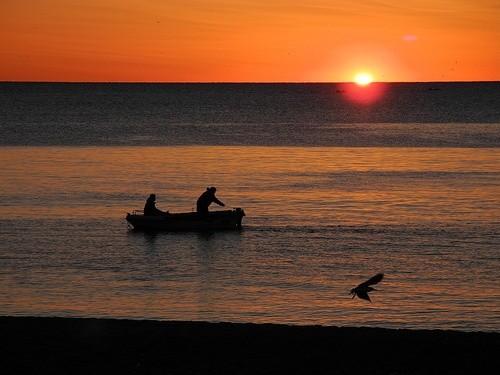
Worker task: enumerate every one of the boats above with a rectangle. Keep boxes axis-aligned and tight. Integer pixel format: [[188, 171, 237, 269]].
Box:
[[126, 204, 245, 235]]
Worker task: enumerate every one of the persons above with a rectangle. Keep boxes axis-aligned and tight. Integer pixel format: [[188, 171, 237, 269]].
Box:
[[196, 186, 225, 213], [144, 194, 161, 216]]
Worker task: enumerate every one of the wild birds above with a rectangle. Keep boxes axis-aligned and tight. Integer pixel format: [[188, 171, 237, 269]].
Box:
[[348, 272, 385, 304]]
